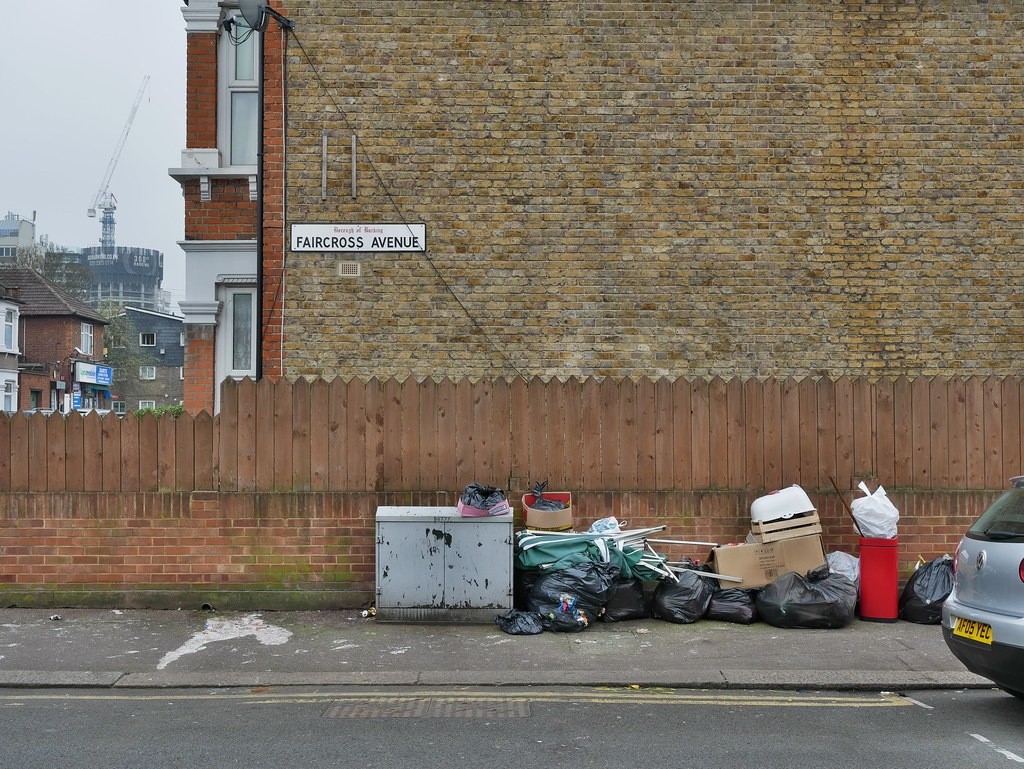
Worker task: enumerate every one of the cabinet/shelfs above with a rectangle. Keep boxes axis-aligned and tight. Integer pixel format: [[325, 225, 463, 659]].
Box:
[[374, 506, 514, 625]]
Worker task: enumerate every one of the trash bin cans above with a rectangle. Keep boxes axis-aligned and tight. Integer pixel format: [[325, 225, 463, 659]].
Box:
[[859, 535, 899, 623]]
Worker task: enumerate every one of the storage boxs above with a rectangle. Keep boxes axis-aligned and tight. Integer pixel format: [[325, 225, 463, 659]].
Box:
[[710, 534, 828, 590], [521, 492, 573, 533]]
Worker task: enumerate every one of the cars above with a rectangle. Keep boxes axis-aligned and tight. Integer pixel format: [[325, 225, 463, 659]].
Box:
[[940, 473, 1024, 701]]
